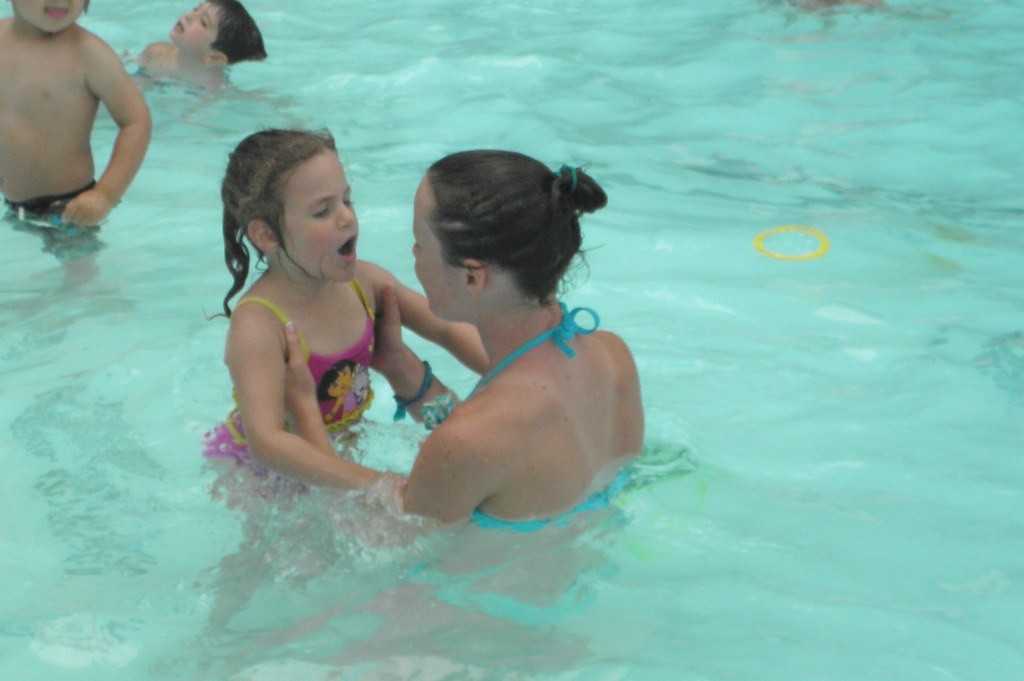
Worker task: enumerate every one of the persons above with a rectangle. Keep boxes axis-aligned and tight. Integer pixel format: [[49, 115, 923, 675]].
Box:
[[283, 149, 647, 521], [140, 0, 268, 88], [0, 0, 152, 228], [219, 128, 489, 494]]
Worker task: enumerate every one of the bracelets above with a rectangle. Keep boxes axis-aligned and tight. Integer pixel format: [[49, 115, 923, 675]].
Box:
[[394, 361, 433, 420]]
